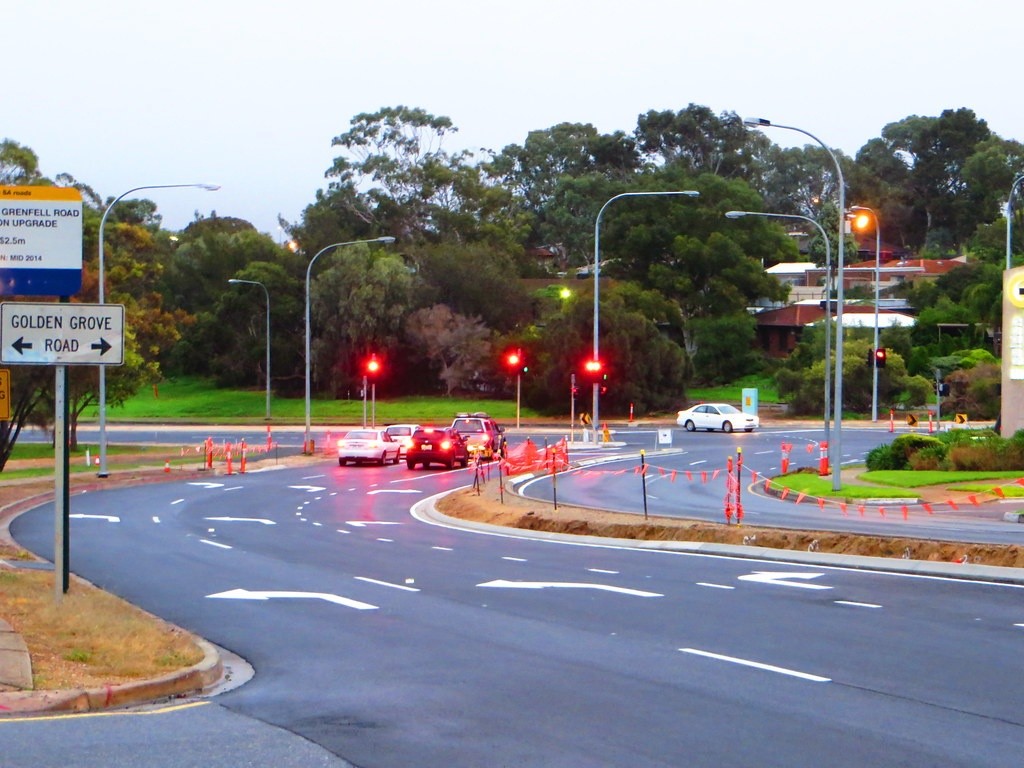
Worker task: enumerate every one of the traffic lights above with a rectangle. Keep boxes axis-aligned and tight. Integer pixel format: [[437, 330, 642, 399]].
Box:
[[602, 373, 607, 381], [598, 386, 608, 396], [876, 349, 887, 369], [574, 388, 579, 398], [865, 348, 873, 367], [522, 366, 529, 373], [508, 354, 521, 374], [586, 358, 602, 376], [366, 359, 382, 381]]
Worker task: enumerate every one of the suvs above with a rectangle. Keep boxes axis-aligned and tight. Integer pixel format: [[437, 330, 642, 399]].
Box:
[[405, 426, 471, 471], [450, 412, 507, 463]]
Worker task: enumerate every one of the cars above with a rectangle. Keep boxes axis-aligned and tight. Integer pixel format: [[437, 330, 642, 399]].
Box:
[[385, 424, 424, 456], [337, 429, 402, 466], [675, 402, 761, 433]]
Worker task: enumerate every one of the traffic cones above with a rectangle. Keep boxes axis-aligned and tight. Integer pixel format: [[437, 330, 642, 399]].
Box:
[[163, 458, 172, 473], [94, 453, 99, 466]]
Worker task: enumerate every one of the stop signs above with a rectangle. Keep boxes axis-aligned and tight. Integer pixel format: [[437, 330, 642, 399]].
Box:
[[1, 302, 126, 368]]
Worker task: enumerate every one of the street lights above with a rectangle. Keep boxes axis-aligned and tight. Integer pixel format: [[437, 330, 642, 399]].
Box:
[[591, 190, 700, 447], [851, 205, 879, 423], [745, 115, 844, 493], [304, 236, 397, 443], [99, 181, 222, 485], [228, 277, 275, 421], [725, 209, 829, 462]]
